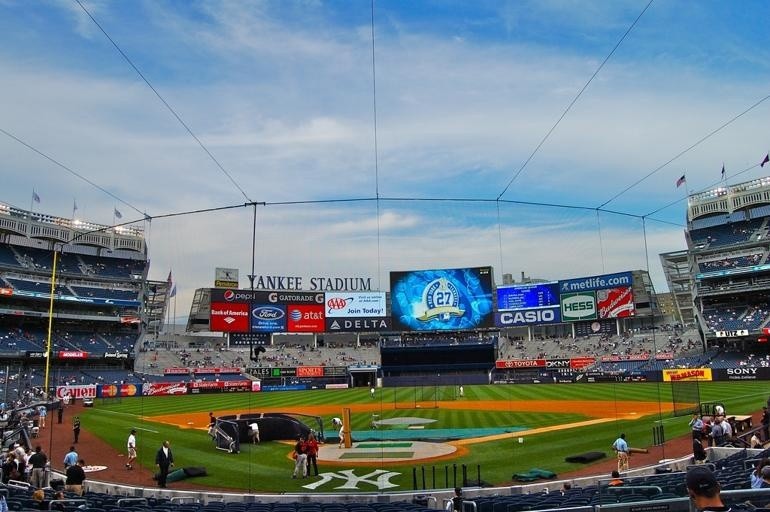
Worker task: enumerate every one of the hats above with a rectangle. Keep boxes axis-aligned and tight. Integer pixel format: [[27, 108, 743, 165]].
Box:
[[688, 467, 716, 489], [455, 488, 462, 496], [763, 466, 770, 474]]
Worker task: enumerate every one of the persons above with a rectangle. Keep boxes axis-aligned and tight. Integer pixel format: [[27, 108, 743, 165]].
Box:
[[0, 225, 770, 512]]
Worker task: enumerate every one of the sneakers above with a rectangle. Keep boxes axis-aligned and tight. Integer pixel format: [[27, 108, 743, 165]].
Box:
[[126, 463, 133, 470]]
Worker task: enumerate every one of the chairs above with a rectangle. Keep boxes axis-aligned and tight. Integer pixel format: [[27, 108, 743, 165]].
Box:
[[687, 214, 769, 371], [0, 241, 253, 387], [590, 359, 766, 372], [380, 335, 495, 349]]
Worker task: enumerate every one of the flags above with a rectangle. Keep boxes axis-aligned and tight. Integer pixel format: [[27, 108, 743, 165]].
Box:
[[74, 199, 78, 212], [677, 176, 685, 188], [33, 193, 40, 203], [761, 155, 769, 168], [721, 166, 724, 180], [115, 210, 122, 219], [145, 215, 150, 222]]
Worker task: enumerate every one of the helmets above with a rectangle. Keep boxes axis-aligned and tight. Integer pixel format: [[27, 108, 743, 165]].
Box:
[[131, 430, 136, 433]]
